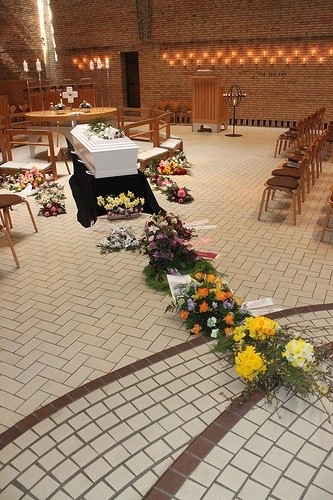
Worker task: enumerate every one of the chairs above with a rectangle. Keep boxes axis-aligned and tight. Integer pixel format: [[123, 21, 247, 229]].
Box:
[[0, 194, 38, 233], [258, 105, 333, 244]]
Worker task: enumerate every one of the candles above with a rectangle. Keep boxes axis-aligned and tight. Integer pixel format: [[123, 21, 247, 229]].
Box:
[[162, 49, 333, 66], [23, 59, 29, 71], [36, 59, 42, 70], [90, 57, 110, 70]]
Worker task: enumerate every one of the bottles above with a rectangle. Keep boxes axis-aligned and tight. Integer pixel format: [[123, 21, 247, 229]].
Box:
[[49, 102, 59, 115]]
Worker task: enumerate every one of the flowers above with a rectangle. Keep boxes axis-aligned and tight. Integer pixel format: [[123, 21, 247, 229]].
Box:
[[142, 151, 195, 204], [141, 214, 224, 292], [216, 316, 333, 424], [166, 267, 255, 337], [96, 226, 144, 254], [97, 191, 145, 220], [1, 167, 67, 218], [87, 121, 125, 139]]
